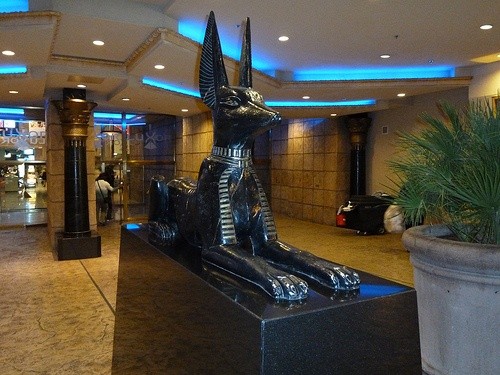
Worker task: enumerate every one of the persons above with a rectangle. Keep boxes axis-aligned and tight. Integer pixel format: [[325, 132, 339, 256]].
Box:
[[399, 183, 426, 252], [93, 170, 123, 229]]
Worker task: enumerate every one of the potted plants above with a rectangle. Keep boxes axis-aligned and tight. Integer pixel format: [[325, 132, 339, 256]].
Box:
[[378, 88, 500, 375]]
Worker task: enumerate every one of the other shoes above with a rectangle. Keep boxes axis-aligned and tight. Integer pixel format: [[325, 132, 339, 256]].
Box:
[[100, 222, 109, 225]]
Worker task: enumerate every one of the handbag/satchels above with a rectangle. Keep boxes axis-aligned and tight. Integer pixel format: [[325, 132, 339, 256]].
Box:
[[103, 197, 111, 204]]
